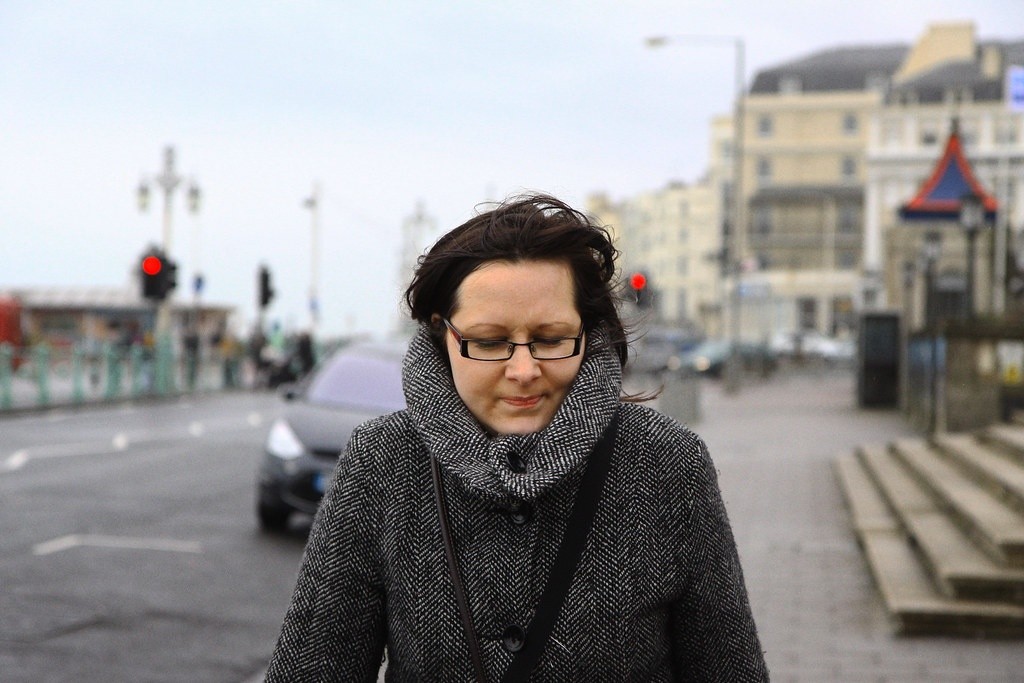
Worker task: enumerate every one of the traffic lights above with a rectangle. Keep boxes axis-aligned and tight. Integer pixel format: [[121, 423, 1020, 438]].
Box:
[[627, 269, 652, 308], [140, 247, 170, 303]]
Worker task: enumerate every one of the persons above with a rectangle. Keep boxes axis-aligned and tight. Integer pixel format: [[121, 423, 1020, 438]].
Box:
[[264, 191, 769, 683]]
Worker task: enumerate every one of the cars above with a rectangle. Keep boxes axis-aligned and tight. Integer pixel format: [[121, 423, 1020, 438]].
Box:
[[255, 336, 409, 531]]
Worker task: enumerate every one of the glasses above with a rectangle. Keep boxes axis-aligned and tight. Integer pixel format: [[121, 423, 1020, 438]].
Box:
[[442, 318, 587, 362]]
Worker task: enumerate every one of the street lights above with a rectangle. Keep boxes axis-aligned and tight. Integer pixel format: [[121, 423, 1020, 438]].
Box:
[[960, 191, 984, 333], [644, 34, 749, 396], [135, 148, 201, 402]]
